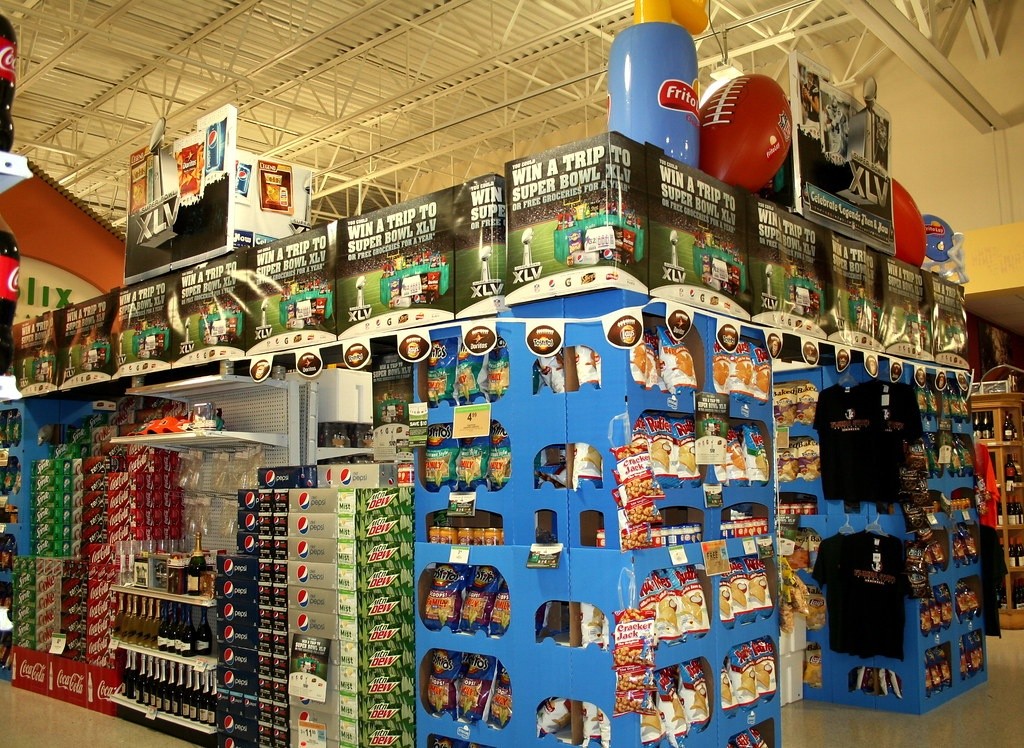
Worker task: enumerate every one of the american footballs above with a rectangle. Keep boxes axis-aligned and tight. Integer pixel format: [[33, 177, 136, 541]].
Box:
[[699, 72, 793, 189], [891, 176, 928, 269]]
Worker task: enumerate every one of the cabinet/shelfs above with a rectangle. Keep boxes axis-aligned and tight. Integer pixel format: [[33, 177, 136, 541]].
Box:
[[972, 392, 1024, 630], [108, 378, 301, 748]]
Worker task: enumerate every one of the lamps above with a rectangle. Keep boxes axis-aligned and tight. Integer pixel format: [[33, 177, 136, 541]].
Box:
[[699, 30, 745, 109]]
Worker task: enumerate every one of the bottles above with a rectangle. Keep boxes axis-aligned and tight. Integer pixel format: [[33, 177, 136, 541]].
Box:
[[995, 578, 1024, 609], [973, 412, 981, 440], [1000, 537, 1024, 567], [127, 652, 137, 698], [113, 594, 212, 657], [188, 531, 206, 594], [151, 658, 217, 725], [144, 656, 153, 705], [122, 650, 131, 695], [1003, 415, 1012, 440], [997, 495, 1024, 524], [1013, 454, 1022, 481], [983, 412, 991, 438], [135, 654, 146, 704], [1006, 453, 1015, 481]]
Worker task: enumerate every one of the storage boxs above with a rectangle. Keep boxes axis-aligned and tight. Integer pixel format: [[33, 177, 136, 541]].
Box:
[[11, 395, 194, 672], [286, 367, 373, 425], [779, 612, 807, 655], [779, 651, 805, 707], [11, 130, 970, 392], [214, 462, 414, 748]]
